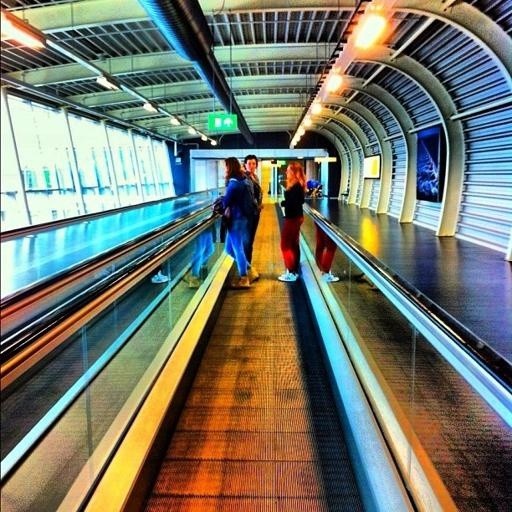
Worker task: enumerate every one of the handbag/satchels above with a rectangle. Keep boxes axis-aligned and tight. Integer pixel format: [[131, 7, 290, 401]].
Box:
[[212, 197, 231, 218]]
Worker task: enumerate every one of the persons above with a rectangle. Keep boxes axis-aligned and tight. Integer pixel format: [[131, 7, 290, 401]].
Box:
[[306, 179, 322, 199], [188, 221, 216, 290], [313, 221, 340, 283], [151, 265, 169, 284], [244, 154, 264, 265], [277, 161, 308, 283], [240, 165, 246, 171], [219, 157, 261, 290]]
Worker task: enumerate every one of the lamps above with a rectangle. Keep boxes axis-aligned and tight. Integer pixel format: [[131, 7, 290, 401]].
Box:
[[97, 76, 124, 93], [1, 8, 46, 52], [289, 0, 386, 149], [143, 101, 218, 146]]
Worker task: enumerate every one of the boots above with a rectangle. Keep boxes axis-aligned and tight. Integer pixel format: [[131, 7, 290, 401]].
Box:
[[185, 265, 208, 287], [229, 266, 260, 288]]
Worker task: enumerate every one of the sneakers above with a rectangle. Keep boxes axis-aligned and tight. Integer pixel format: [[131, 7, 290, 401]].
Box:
[[151, 270, 169, 284], [277, 270, 299, 282], [323, 272, 339, 282]]
